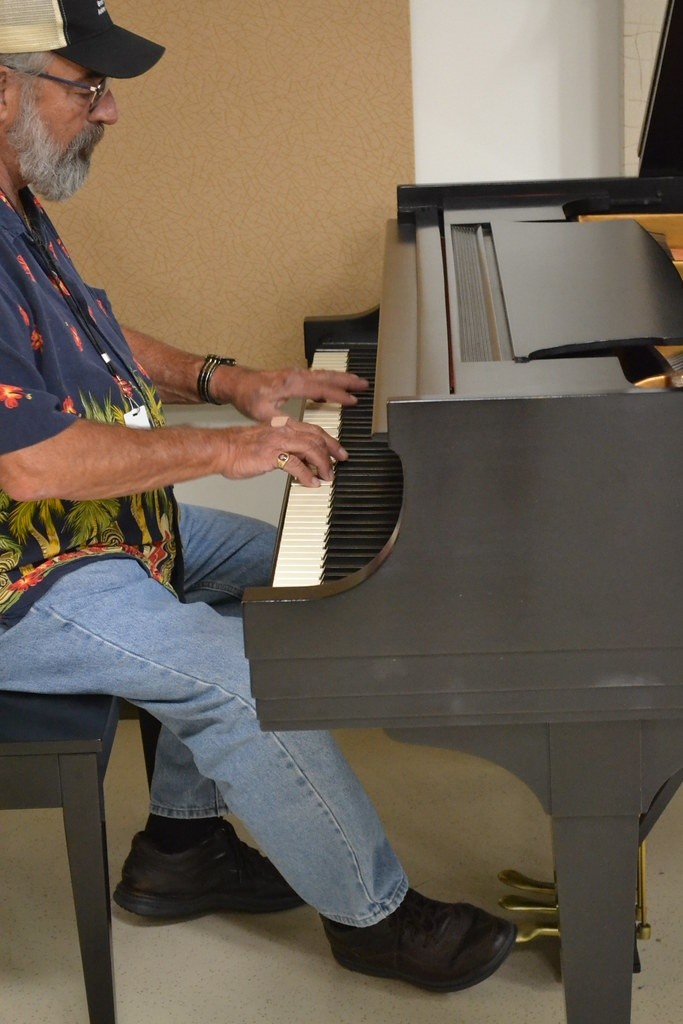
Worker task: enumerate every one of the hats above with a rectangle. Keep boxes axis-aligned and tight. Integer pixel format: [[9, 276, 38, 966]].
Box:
[[0, 0, 166, 78]]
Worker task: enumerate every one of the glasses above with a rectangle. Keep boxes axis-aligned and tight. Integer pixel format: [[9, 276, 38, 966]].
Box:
[[3, 64, 111, 114]]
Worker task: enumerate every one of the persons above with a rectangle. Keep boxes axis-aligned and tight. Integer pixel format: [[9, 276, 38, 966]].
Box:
[[0, 0, 518, 993]]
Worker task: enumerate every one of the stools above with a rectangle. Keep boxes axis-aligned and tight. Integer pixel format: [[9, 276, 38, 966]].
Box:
[[0, 690, 165, 1024]]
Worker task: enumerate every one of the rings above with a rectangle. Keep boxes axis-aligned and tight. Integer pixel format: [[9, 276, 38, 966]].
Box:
[[271, 416, 290, 427], [277, 452, 289, 469]]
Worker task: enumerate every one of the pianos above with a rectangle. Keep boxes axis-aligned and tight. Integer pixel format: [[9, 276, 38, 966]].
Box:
[[240, 0, 683, 1024]]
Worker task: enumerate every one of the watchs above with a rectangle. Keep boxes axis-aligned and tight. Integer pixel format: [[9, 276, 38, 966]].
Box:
[[197, 355, 236, 406]]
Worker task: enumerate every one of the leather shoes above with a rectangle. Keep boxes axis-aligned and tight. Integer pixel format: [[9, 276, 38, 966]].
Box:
[[319, 888, 517, 993], [112, 813, 307, 917]]
